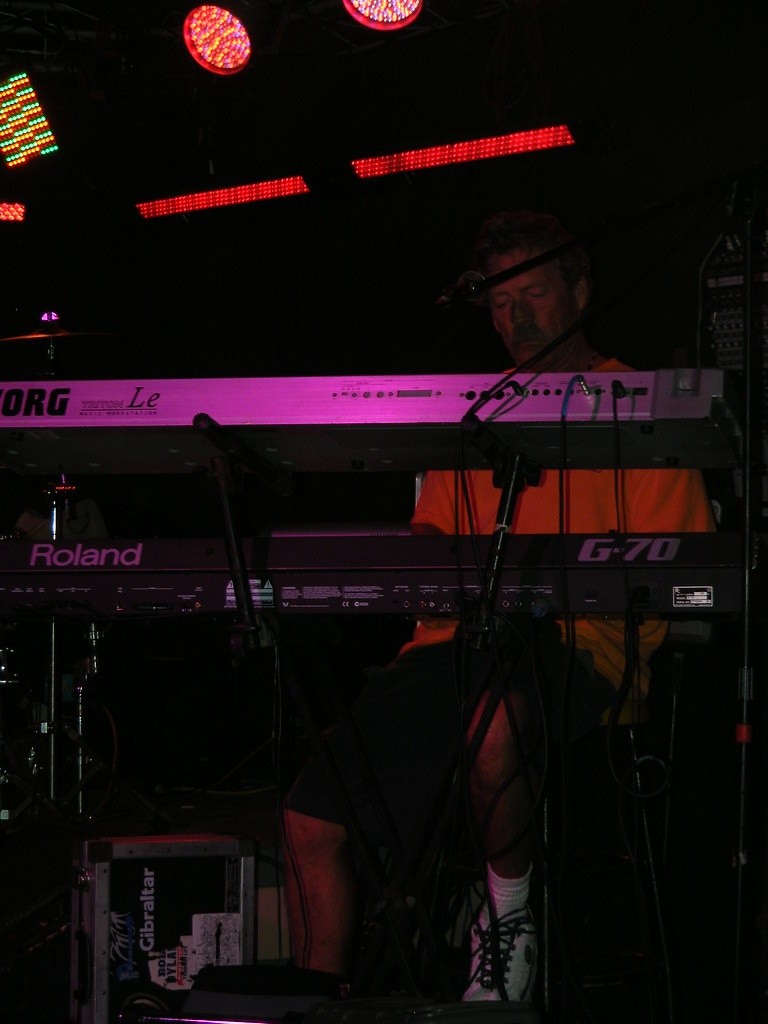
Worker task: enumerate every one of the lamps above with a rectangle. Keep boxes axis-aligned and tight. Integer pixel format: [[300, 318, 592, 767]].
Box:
[[340, 0, 424, 32], [182, 0, 283, 77]]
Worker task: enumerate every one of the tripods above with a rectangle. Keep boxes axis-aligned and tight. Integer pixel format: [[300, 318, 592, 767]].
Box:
[[351, 455, 599, 1023]]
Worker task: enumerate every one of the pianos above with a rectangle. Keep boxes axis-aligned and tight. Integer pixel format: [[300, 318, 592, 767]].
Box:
[[0, 364, 768, 1023]]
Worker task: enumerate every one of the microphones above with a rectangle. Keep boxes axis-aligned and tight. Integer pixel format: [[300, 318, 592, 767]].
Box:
[[436, 271, 489, 310], [192, 414, 282, 482], [464, 415, 526, 493]]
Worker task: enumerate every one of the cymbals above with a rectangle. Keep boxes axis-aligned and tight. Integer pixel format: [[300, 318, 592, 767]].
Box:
[[0, 325, 118, 343]]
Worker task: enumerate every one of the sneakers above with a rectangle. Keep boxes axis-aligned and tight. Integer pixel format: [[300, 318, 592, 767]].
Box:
[[462, 903, 538, 1003]]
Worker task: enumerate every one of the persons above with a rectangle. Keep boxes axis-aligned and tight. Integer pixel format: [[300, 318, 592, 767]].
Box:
[[281, 214, 717, 1001]]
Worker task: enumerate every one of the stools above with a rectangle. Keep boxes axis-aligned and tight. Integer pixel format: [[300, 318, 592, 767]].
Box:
[[70, 836, 257, 1023], [536, 698, 658, 1022]]
